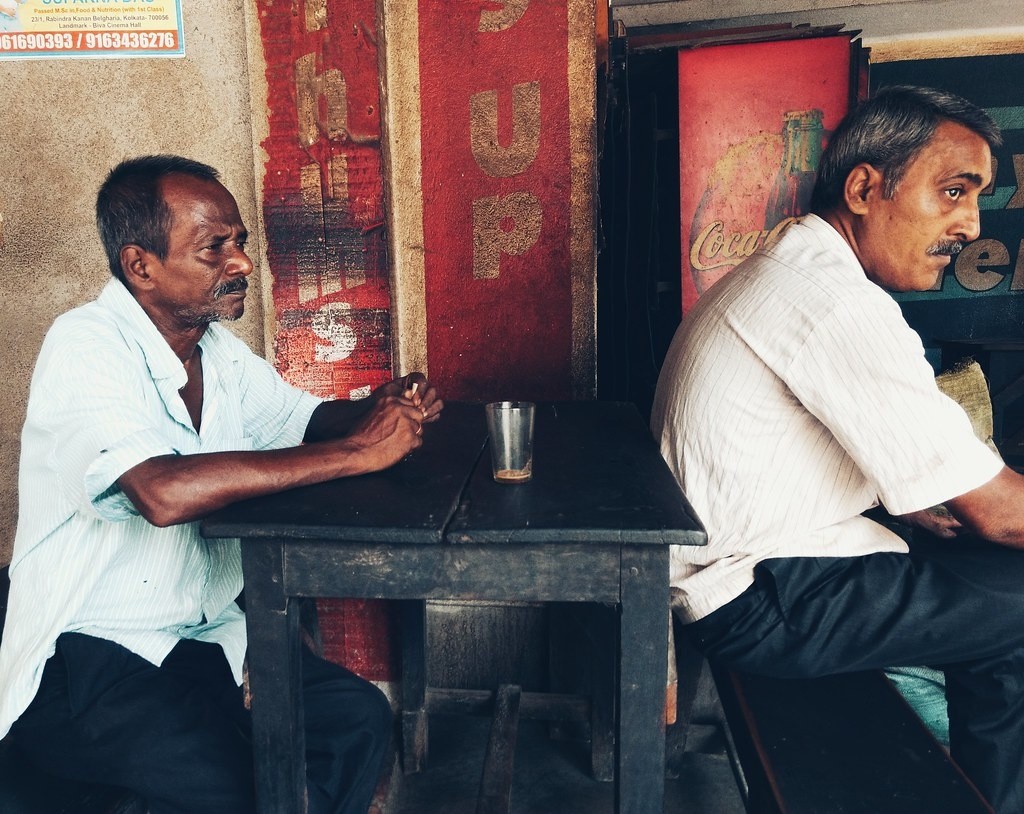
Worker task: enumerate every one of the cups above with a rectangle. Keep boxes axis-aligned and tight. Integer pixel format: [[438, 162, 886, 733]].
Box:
[[485, 401, 535, 483]]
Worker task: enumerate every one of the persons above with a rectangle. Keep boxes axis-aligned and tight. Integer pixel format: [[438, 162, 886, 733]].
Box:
[[648, 84, 1024, 814], [1, 155, 445, 814]]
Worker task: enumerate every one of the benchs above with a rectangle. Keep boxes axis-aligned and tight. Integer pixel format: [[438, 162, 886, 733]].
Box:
[[704, 656, 996, 814]]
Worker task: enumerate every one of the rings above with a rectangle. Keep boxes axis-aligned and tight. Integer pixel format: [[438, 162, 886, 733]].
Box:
[[414, 421, 422, 433]]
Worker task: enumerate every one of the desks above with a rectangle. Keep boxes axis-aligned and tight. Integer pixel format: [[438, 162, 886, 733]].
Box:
[[197, 398, 709, 814]]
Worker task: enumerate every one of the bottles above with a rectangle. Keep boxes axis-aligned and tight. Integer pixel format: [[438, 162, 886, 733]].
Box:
[[764, 108, 824, 244]]
